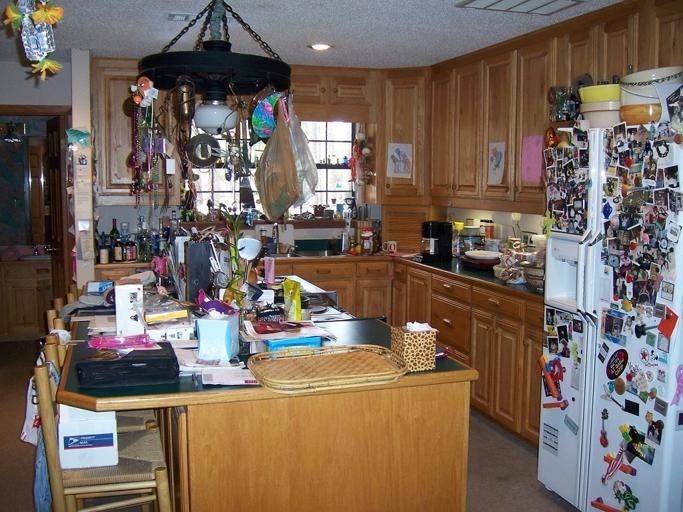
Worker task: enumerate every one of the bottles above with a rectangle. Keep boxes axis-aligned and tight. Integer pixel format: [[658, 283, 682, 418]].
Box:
[[328, 155, 348, 166], [329, 198, 357, 221], [361, 232, 373, 255], [98, 210, 186, 264], [480, 219, 494, 240]]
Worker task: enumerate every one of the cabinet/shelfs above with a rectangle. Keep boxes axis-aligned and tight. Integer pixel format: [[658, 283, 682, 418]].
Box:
[[558, 0, 683, 94], [378, 66, 427, 207], [288, 64, 377, 124], [429, 21, 557, 215], [471, 284, 541, 447], [90, 56, 180, 207], [390, 259, 472, 368], [0, 263, 53, 342], [292, 261, 394, 326]]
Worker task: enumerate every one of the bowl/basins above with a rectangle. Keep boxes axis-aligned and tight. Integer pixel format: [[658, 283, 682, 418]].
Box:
[[579, 65, 683, 128]]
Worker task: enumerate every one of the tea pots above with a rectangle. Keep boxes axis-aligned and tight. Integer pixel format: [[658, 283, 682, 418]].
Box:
[[313, 205, 326, 217]]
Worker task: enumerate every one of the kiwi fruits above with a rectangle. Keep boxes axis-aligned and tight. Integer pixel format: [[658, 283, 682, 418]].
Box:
[[615, 377, 625, 395]]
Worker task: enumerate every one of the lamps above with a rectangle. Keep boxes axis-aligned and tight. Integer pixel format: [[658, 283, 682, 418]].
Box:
[[135, 1, 293, 138]]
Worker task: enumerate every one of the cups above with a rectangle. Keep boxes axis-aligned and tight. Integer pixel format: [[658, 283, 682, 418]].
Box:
[[382, 240, 397, 254]]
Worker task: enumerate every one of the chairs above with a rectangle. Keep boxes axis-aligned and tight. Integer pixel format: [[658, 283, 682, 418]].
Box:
[[31, 280, 173, 511]]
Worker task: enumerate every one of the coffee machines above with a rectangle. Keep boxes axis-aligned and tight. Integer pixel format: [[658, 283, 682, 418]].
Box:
[[421, 221, 452, 263]]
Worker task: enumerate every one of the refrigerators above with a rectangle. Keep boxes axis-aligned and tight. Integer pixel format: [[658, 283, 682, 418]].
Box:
[[537, 127, 682, 512]]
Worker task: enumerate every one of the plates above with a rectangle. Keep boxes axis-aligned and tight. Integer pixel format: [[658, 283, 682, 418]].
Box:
[[462, 249, 501, 269]]
